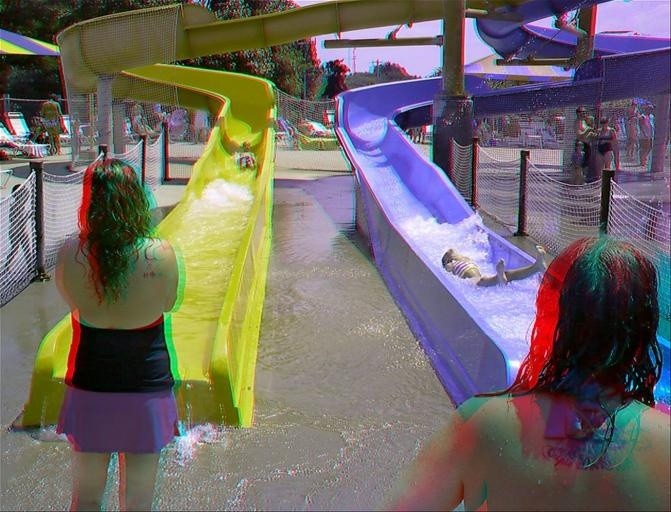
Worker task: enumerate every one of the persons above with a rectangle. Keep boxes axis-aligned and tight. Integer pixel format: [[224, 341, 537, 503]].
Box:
[[543, 99, 661, 182], [235, 141, 255, 170], [377, 238, 671, 511], [32, 115, 44, 139], [42, 93, 63, 154], [55, 159, 179, 511], [129, 100, 207, 145], [443, 246, 545, 290]]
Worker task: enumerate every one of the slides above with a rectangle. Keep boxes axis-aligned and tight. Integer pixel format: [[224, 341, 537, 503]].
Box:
[[12, 1, 500, 428], [335, 1, 670, 410]]
